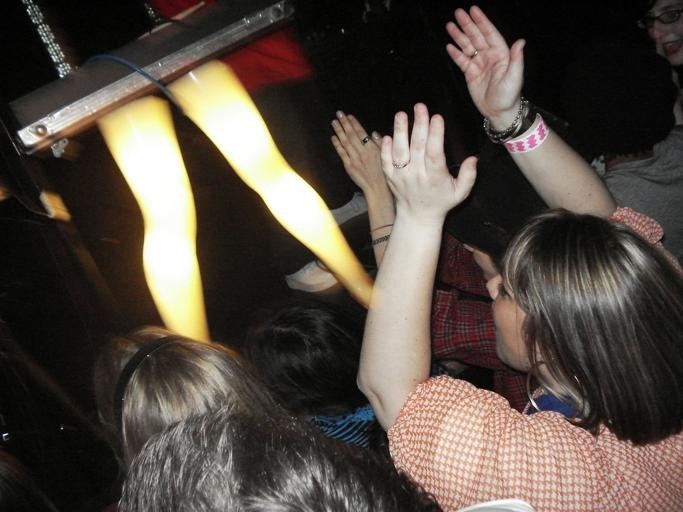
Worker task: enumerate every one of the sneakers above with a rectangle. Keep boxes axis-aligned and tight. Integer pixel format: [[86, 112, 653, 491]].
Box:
[[328, 190, 370, 232], [282, 258, 338, 294]]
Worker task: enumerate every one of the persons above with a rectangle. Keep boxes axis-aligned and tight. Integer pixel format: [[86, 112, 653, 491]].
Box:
[[0, 0, 683, 510]]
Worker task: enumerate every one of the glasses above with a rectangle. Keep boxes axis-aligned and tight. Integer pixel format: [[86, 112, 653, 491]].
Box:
[[635, 8, 683, 29]]
